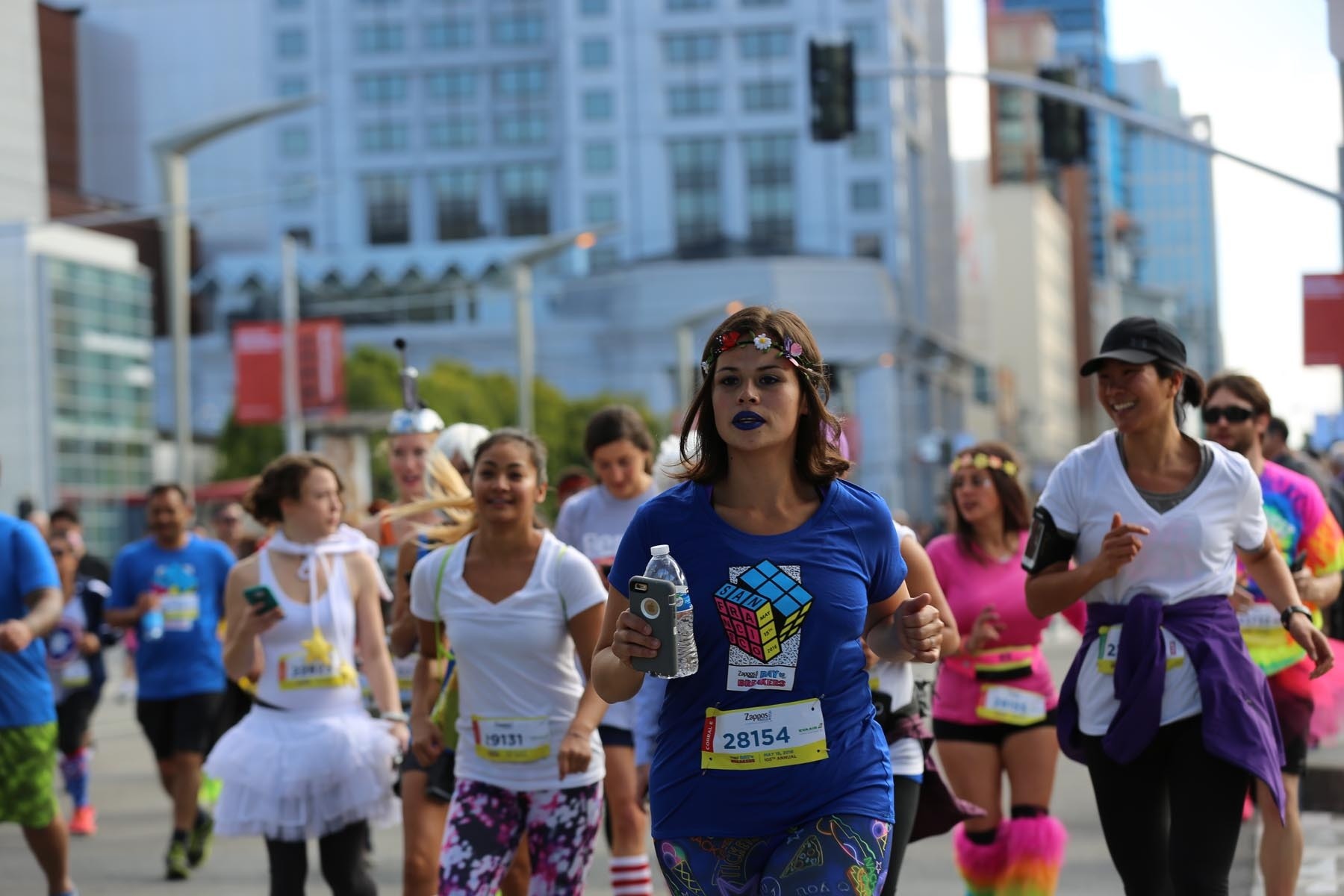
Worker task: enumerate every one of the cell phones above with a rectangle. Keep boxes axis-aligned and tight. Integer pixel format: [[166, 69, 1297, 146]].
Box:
[[242, 582, 285, 619], [1291, 548, 1308, 574], [628, 575, 677, 676], [1020, 512, 1045, 570]]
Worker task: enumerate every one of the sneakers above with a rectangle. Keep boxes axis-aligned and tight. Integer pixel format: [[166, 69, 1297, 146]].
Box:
[[70, 806, 98, 836], [185, 814, 216, 869], [166, 841, 191, 880]]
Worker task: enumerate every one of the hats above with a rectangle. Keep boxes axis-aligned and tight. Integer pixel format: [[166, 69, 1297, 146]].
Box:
[[1080, 316, 1186, 376]]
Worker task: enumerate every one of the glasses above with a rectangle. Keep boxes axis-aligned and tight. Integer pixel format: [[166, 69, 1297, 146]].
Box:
[[1202, 406, 1253, 425]]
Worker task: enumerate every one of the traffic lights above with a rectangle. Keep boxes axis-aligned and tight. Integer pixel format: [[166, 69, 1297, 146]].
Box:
[[807, 36, 854, 140]]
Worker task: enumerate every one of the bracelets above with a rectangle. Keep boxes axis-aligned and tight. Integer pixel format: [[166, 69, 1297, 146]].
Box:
[[1280, 605, 1313, 631], [380, 711, 410, 723]]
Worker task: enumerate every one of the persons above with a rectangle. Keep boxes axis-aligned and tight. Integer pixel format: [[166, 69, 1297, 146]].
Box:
[[817, 415, 961, 896], [916, 442, 1089, 896], [1199, 373, 1344, 896], [0, 399, 668, 896], [1022, 316, 1333, 895], [587, 307, 943, 896]]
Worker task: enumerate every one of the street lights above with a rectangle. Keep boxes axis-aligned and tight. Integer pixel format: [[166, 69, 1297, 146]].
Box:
[[505, 220, 615, 440], [151, 87, 331, 511], [676, 300, 745, 425]]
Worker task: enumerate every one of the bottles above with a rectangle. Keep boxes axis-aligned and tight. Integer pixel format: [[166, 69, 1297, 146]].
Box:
[[141, 609, 163, 642], [642, 546, 698, 680]]
